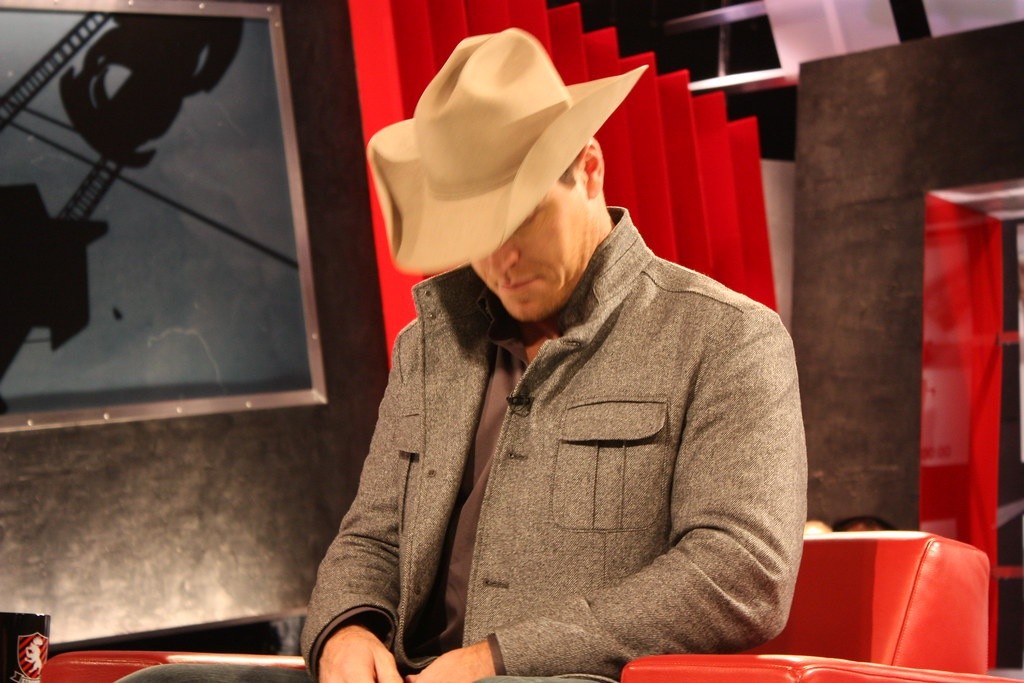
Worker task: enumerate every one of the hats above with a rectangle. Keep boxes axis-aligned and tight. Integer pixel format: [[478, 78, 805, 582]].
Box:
[[367, 28, 649, 275]]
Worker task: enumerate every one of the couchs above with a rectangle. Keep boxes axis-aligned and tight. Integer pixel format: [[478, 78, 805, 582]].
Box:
[[38, 526, 1024, 683]]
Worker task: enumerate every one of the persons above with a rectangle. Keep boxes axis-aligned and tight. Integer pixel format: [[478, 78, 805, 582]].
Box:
[[300, 29, 807, 683]]
